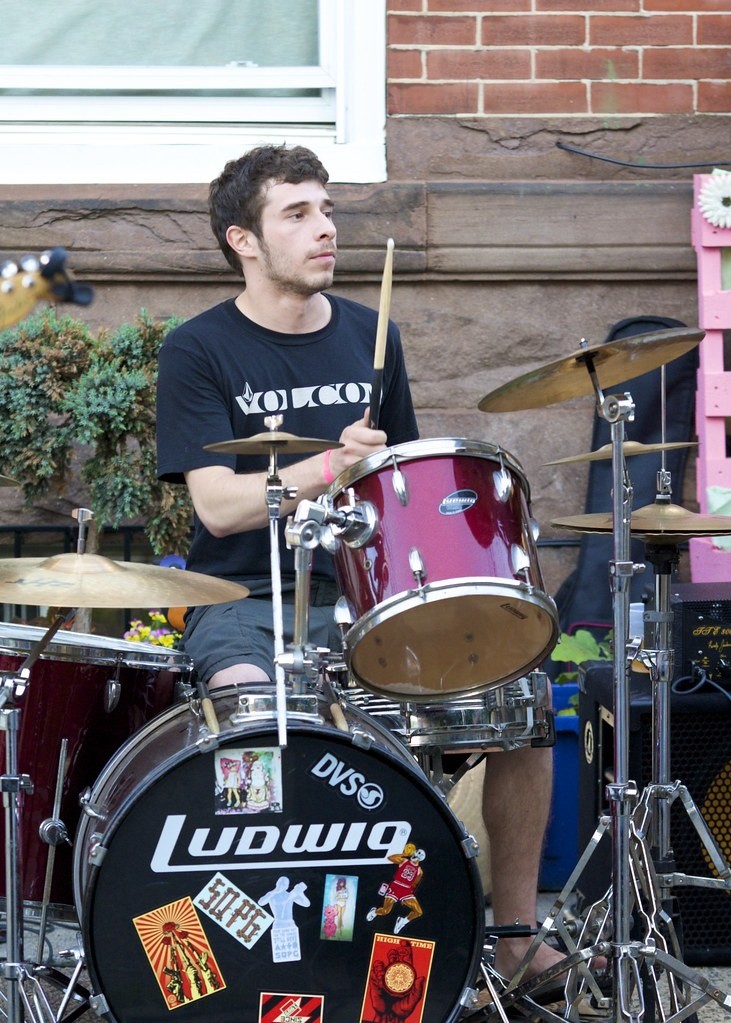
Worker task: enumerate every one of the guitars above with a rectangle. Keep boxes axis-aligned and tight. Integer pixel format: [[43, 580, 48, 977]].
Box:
[[0, 247, 94, 334]]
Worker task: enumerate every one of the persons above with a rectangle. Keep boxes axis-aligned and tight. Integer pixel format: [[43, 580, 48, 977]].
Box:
[[156, 145, 608, 1003]]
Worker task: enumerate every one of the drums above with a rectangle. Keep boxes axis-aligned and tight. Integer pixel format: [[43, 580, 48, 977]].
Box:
[[342, 670, 541, 754], [71, 681, 486, 1023], [0, 621, 194, 923], [325, 436, 560, 704]]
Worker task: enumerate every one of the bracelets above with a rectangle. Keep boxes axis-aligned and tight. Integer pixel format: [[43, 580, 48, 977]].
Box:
[[323, 448, 337, 483]]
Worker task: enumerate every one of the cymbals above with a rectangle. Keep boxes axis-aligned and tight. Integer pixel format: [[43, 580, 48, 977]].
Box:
[[0, 552, 250, 608], [477, 327, 706, 413], [202, 432, 346, 454], [549, 503, 731, 546], [541, 441, 703, 466]]
